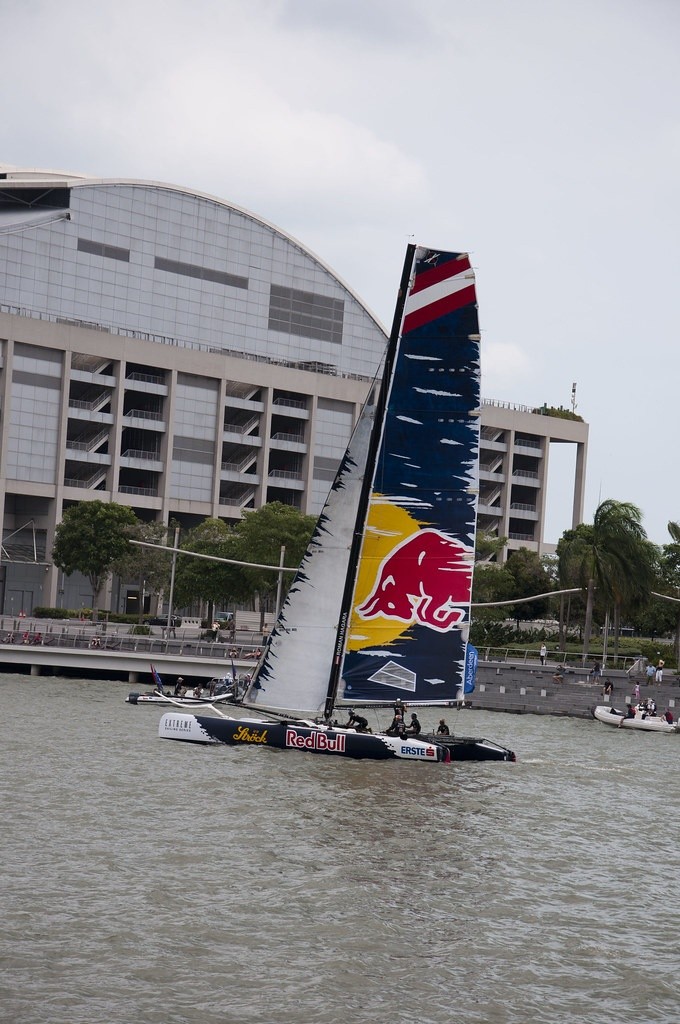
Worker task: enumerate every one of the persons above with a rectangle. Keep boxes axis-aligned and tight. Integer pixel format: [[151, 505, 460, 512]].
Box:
[[632, 681, 640, 700], [211, 621, 220, 643], [551, 665, 566, 684], [2, 630, 43, 646], [590, 661, 600, 685], [603, 678, 613, 701], [174, 674, 252, 700], [262, 623, 271, 645], [644, 660, 664, 687], [346, 697, 450, 737], [661, 708, 673, 724], [540, 643, 547, 666], [618, 704, 636, 728], [229, 645, 260, 659]]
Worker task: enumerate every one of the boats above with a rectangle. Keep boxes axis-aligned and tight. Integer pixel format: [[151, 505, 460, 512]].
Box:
[[125, 693, 232, 703], [593, 705, 680, 733]]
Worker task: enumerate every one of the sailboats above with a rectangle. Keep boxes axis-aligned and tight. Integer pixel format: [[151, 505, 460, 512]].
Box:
[[159, 242, 517, 762]]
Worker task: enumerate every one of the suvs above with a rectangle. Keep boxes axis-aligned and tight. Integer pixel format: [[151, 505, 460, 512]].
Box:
[[213, 613, 234, 626]]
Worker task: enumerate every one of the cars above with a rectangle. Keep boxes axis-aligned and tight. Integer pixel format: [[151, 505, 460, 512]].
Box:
[[152, 614, 183, 625]]
